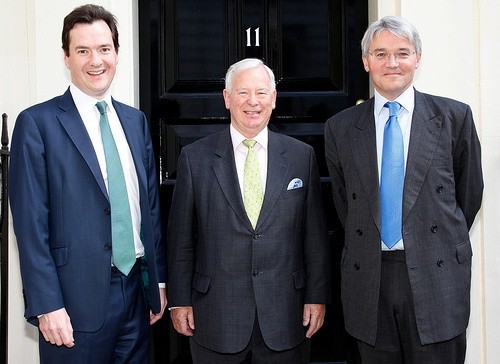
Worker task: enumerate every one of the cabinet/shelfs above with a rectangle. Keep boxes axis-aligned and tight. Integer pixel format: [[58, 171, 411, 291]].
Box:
[[137, 0, 372, 364]]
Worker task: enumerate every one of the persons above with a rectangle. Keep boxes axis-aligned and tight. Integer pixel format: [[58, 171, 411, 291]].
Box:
[[5, 4, 168, 364], [324, 16, 484, 364], [165, 57, 328, 364]]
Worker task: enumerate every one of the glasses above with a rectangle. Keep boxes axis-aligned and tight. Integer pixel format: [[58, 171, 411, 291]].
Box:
[[367, 49, 416, 61]]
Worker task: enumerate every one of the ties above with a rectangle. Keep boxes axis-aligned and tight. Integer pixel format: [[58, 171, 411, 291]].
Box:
[[380, 100, 406, 250], [95, 100, 136, 277], [241, 140, 265, 230]]
